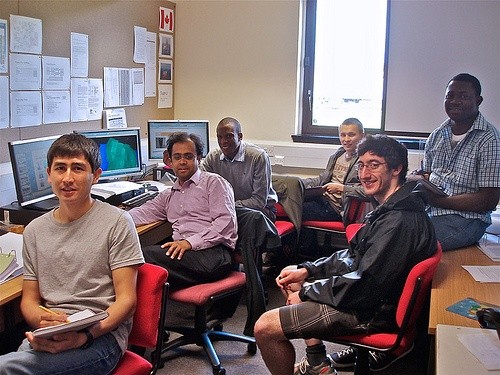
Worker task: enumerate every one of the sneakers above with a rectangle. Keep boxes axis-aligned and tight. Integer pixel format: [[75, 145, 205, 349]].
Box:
[[294, 354, 339, 375], [368, 334, 414, 371], [326, 346, 370, 367]]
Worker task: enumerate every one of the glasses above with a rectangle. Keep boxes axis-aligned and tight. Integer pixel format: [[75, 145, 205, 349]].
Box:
[[354, 162, 386, 171]]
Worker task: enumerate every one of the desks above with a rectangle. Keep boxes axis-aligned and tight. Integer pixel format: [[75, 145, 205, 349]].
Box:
[[428, 245, 500, 375], [0, 203, 174, 353]]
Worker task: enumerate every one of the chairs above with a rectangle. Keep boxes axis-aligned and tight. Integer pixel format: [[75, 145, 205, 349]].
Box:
[[109, 175, 442, 375]]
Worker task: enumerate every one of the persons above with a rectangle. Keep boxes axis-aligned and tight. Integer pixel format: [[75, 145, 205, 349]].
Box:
[[300, 118, 375, 222], [0, 131, 146, 375], [254, 133, 438, 375], [406, 73, 500, 251], [201, 117, 277, 223], [128, 131, 238, 356]]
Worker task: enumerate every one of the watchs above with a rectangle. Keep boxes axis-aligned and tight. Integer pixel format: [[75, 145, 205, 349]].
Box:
[[77, 327, 94, 349]]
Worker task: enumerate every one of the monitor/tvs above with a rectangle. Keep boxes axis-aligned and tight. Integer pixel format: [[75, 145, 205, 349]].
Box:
[[8, 134, 57, 207], [147, 119, 210, 160], [73, 127, 143, 183]]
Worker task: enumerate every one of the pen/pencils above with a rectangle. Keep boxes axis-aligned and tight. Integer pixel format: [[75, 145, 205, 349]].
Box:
[[39, 305, 71, 322], [421, 160, 424, 175], [279, 277, 290, 291]]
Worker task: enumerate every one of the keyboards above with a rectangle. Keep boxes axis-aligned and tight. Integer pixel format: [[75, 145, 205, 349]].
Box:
[[122, 192, 160, 209]]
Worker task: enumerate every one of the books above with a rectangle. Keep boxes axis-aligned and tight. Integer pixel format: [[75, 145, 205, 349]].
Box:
[[31, 312, 110, 337]]
